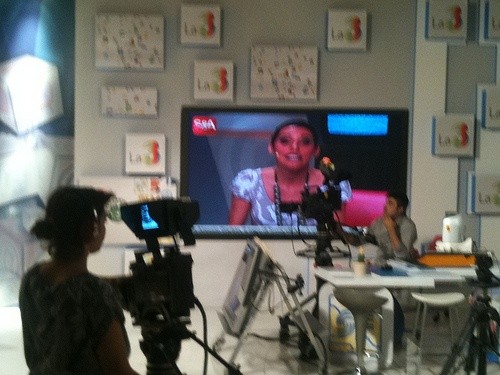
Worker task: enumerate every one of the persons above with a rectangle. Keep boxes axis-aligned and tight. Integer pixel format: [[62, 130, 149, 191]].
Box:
[[19, 185, 140, 375], [229, 120, 353, 225], [141, 205, 159, 230], [336, 193, 417, 262]]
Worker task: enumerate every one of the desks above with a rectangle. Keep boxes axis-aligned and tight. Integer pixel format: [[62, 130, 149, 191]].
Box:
[[314, 266, 466, 287]]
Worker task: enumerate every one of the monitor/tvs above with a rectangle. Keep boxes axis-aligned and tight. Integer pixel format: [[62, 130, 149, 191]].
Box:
[[180, 104, 413, 239]]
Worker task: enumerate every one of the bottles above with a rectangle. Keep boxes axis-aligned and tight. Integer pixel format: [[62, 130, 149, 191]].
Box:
[[358, 245, 365, 262]]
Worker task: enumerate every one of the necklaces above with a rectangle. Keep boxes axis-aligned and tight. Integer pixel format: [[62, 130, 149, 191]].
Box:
[[274, 167, 309, 226]]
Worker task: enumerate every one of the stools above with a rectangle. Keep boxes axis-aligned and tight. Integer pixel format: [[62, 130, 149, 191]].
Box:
[[331, 288, 389, 375], [410, 291, 466, 375]]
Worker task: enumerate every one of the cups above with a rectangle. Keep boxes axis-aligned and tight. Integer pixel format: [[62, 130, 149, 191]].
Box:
[[351, 261, 368, 274]]
